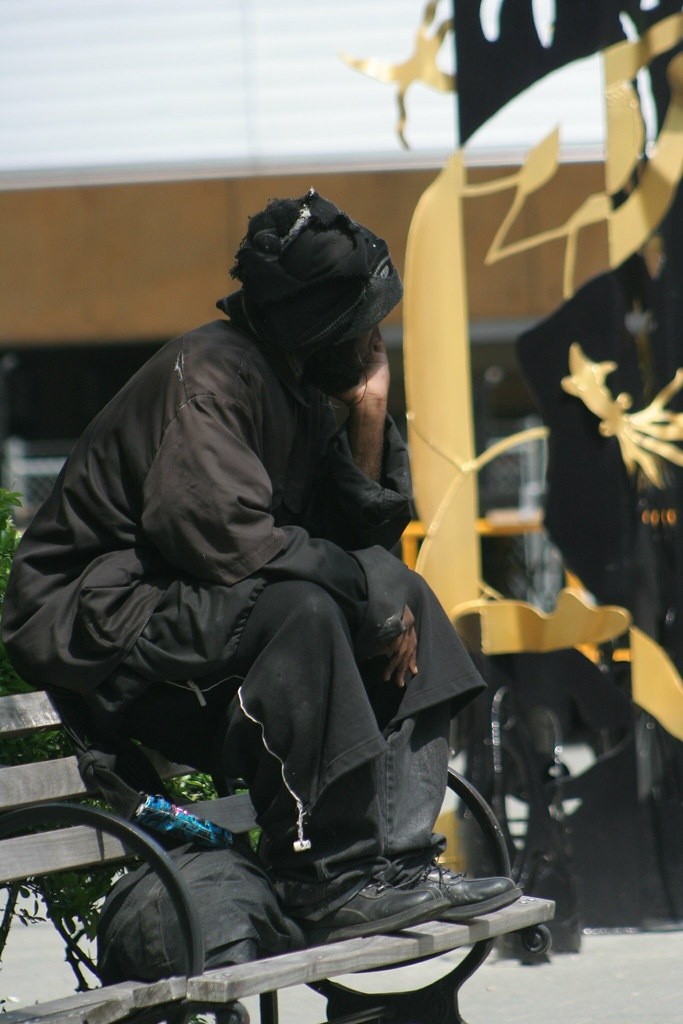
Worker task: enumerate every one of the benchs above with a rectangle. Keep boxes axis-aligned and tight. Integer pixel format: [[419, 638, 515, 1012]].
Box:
[[0, 647, 556, 1024]]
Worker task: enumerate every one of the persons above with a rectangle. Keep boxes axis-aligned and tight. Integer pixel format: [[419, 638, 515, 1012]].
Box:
[[1, 191, 523, 942]]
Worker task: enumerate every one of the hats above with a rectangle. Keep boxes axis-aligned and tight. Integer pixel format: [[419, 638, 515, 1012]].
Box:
[[215, 188, 403, 350]]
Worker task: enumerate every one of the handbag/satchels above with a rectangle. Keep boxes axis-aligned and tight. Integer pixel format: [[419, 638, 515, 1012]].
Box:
[[96, 846, 306, 988]]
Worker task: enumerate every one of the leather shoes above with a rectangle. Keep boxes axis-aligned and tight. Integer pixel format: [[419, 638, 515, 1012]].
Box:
[[403, 860, 522, 920], [309, 879, 451, 946]]
